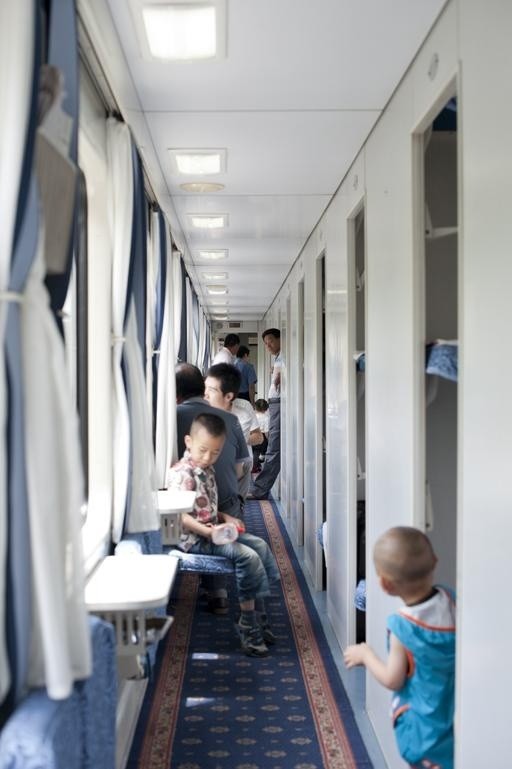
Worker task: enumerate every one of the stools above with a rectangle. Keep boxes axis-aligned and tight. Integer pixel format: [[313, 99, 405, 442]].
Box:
[[166, 549, 232, 575]]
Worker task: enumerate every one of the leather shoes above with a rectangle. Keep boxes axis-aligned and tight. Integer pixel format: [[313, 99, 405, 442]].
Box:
[[207, 597, 230, 614], [247, 495, 263, 499], [251, 463, 262, 473]]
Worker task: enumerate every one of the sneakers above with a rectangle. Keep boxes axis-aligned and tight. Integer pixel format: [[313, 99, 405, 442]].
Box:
[[233, 612, 271, 656], [255, 610, 276, 643]]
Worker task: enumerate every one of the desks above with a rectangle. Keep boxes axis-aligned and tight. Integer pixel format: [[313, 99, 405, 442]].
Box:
[[147, 487, 199, 547], [85, 550, 178, 683]]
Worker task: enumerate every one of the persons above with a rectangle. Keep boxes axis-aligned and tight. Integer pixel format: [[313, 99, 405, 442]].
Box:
[[174, 362, 249, 615], [174, 328, 286, 518], [164, 412, 281, 658], [342, 525, 454, 769]]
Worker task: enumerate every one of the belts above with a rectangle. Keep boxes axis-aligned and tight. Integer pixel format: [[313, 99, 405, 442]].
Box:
[[268, 398, 280, 403]]
